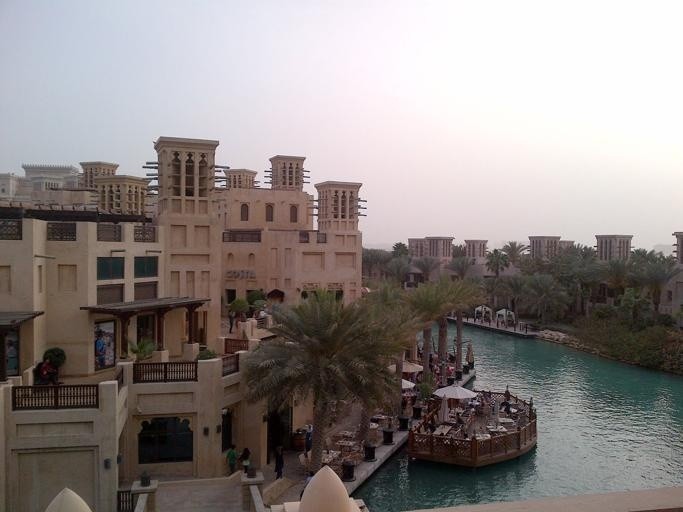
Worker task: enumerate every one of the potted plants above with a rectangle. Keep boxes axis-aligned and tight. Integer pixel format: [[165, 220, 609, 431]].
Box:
[[232, 299, 248, 328], [128, 339, 156, 380]]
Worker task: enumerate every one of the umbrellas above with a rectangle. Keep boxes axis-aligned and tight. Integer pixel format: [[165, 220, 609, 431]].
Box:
[[387, 359, 424, 373]]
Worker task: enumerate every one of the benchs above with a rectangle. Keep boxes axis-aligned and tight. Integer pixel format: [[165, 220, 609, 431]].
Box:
[[34, 362, 51, 392]]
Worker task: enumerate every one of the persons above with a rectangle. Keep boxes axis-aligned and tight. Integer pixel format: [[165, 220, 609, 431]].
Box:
[[223, 444, 240, 474], [400, 347, 511, 442], [4, 340, 19, 376], [272, 447, 285, 480], [237, 447, 252, 472], [305, 424, 312, 451], [39, 357, 63, 385], [226, 309, 234, 333]]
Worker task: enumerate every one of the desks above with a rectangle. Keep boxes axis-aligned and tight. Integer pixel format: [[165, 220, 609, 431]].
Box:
[[299, 363, 524, 476]]
